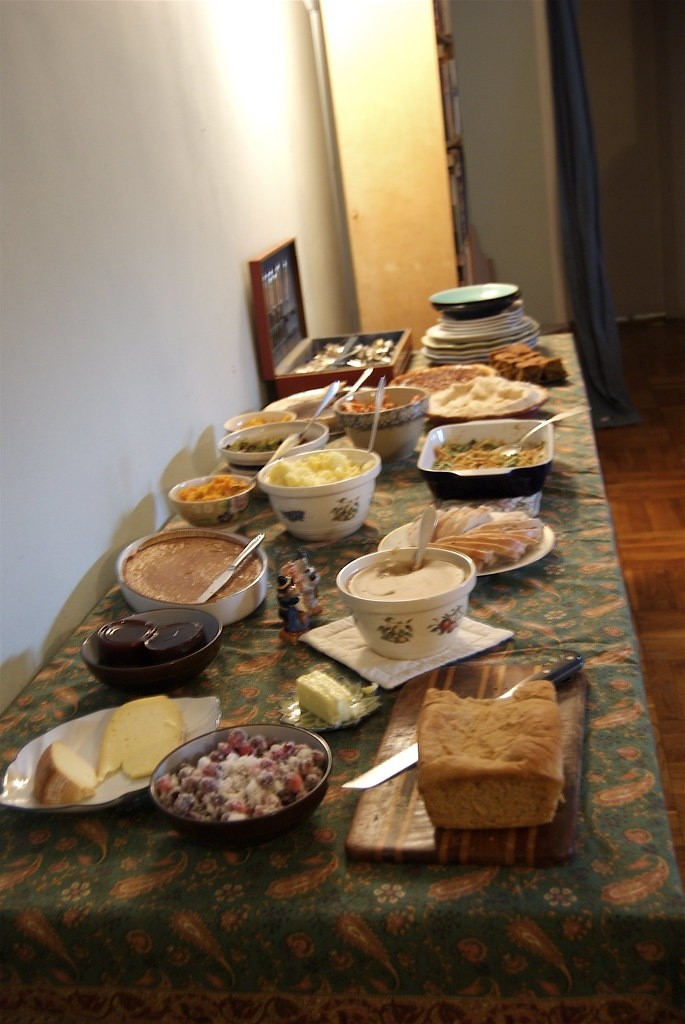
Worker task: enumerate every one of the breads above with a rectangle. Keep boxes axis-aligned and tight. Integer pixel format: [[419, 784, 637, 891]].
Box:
[[410, 505, 545, 570], [417, 677, 562, 830], [96, 694, 185, 783], [33, 741, 98, 806], [487, 345, 566, 382]]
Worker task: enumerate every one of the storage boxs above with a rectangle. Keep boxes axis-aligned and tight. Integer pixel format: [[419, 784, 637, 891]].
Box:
[[247, 236, 413, 404]]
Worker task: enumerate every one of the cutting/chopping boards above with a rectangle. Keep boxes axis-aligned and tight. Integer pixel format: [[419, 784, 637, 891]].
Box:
[[346, 663, 588, 863]]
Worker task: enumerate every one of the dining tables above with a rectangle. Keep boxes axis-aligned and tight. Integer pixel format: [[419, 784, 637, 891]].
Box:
[[0, 333, 685, 1023]]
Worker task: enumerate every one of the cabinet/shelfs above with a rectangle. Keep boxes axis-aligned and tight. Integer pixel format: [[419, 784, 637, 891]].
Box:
[[317, 0, 475, 353]]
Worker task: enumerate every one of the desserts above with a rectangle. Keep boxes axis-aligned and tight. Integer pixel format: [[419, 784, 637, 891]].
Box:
[[295, 671, 354, 726]]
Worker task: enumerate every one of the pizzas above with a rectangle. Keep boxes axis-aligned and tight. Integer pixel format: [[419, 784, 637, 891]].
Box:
[[388, 363, 496, 396]]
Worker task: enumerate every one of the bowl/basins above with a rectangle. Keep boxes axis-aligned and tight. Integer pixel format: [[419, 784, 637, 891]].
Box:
[[150, 724, 332, 849], [416, 420, 552, 497], [333, 387, 428, 462], [224, 411, 296, 434], [219, 419, 329, 498], [256, 449, 382, 541], [115, 528, 269, 626], [78, 607, 221, 690], [336, 548, 477, 659], [168, 475, 255, 528]]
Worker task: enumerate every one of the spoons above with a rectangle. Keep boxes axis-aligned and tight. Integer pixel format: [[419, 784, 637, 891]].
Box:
[[493, 404, 589, 455]]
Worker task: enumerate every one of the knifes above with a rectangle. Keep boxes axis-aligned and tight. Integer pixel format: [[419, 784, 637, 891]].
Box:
[[196, 532, 265, 604], [342, 652, 581, 788]]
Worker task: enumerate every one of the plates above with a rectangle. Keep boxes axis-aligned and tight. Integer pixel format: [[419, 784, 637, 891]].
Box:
[[386, 365, 498, 398], [261, 387, 372, 435], [420, 284, 541, 363], [421, 382, 549, 427], [378, 521, 556, 576], [0, 697, 220, 811]]
